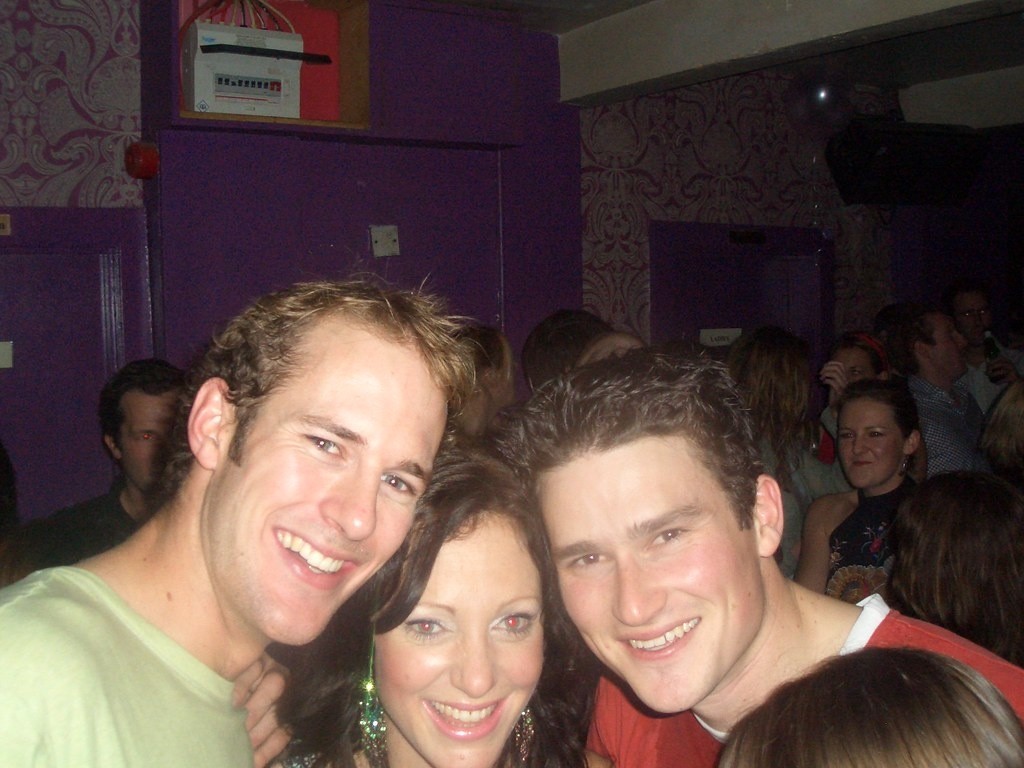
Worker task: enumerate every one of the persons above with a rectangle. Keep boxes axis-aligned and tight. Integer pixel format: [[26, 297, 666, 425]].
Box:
[[234, 345, 1024, 768], [15, 356, 183, 582], [444, 321, 518, 471], [281, 425, 612, 768], [717, 645, 1024, 768], [520, 268, 1024, 664], [0, 269, 479, 768]]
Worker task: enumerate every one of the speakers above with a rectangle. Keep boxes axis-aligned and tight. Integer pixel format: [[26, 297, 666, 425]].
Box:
[[825, 120, 987, 208]]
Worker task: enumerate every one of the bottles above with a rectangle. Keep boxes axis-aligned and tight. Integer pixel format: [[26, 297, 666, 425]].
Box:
[[983, 336, 1004, 385]]
